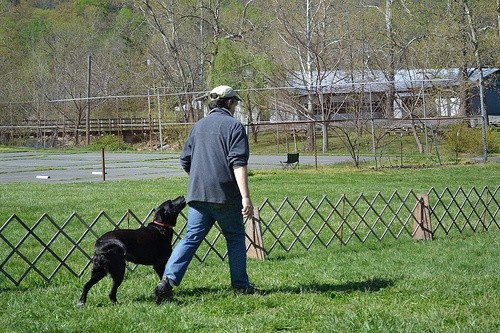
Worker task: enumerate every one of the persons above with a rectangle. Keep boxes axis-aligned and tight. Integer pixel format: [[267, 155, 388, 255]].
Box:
[[153, 84, 265, 305]]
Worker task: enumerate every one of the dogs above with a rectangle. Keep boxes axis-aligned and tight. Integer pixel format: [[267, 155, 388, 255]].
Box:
[[77, 194, 187, 305]]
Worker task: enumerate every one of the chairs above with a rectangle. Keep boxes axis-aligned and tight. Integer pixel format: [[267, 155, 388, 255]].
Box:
[[280, 152, 300, 170]]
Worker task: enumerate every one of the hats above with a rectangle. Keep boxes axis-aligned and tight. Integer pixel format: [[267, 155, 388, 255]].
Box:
[[210, 85, 241, 102]]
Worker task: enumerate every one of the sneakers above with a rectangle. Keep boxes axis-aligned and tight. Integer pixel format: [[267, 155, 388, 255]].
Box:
[[154, 280, 174, 307], [233, 285, 266, 297]]
